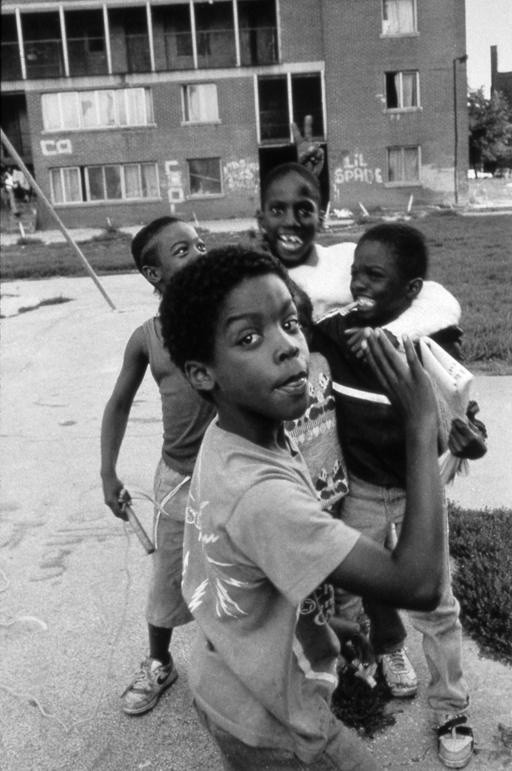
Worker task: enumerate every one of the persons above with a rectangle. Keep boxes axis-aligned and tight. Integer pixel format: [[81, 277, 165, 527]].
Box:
[[96, 110, 328, 716], [252, 162, 463, 370], [307, 220, 490, 769], [156, 243, 455, 771], [282, 280, 386, 698]]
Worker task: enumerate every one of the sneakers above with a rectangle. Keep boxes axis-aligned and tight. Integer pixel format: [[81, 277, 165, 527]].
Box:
[[376, 647, 419, 697], [430, 711, 474, 768], [120, 652, 178, 715]]
